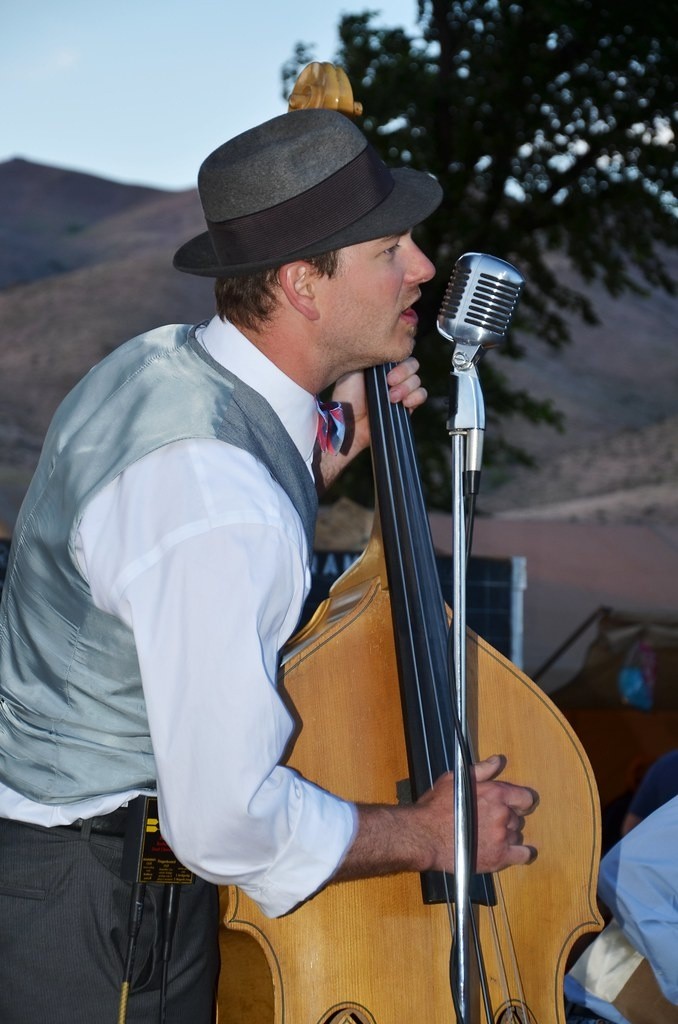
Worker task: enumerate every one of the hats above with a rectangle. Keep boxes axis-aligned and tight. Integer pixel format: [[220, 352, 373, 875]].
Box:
[[172, 108, 443, 278]]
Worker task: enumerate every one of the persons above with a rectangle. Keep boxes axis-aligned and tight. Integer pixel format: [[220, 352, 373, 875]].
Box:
[[597, 749, 678, 1006], [0, 109, 533, 1024]]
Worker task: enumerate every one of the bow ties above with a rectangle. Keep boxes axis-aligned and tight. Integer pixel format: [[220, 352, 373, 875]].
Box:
[[314, 393, 346, 456]]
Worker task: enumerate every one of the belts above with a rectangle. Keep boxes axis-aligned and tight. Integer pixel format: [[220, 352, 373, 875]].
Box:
[[56, 807, 128, 839]]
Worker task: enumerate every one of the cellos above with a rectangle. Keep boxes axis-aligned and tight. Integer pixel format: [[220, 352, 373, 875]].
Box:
[[217, 61, 605, 1024]]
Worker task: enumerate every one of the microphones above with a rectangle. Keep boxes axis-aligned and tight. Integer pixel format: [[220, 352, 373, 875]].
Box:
[[437, 253, 527, 493]]
